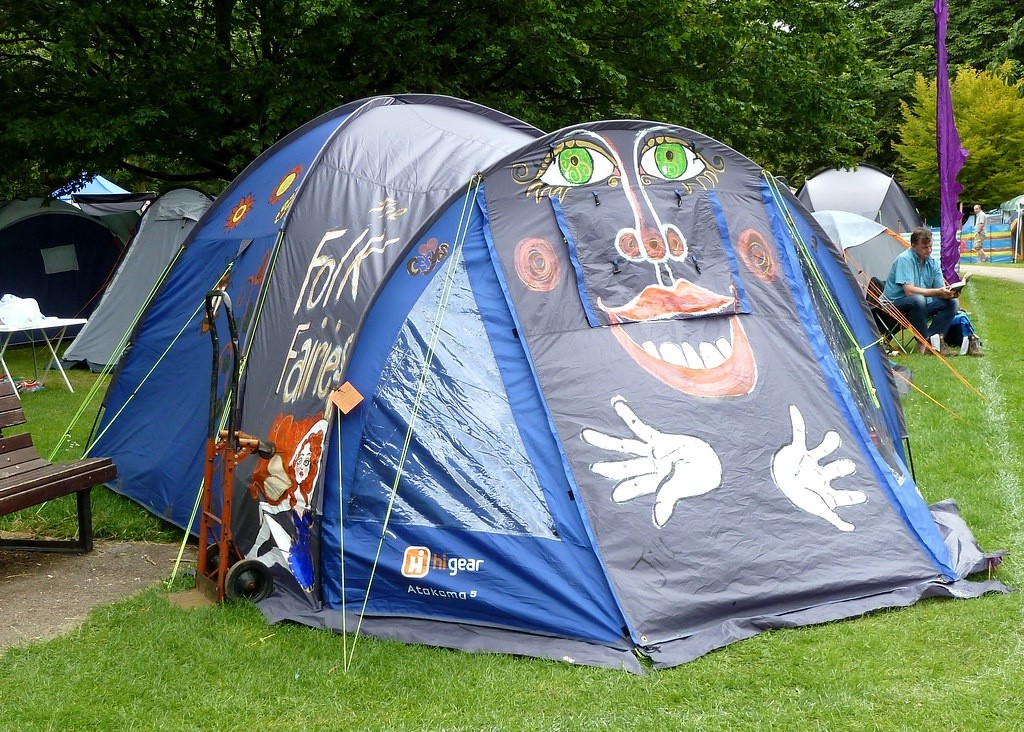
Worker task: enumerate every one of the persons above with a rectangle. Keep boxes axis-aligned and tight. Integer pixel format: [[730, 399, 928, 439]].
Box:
[[876, 226, 961, 357], [974, 205, 989, 264]]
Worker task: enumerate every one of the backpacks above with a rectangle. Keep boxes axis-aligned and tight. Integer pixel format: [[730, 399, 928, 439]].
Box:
[[944, 310, 975, 356]]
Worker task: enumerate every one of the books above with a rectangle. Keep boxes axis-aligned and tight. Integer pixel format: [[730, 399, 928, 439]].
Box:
[[948, 273, 974, 298]]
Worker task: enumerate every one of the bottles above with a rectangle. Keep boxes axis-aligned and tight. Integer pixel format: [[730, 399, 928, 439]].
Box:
[[4, 376, 45, 393]]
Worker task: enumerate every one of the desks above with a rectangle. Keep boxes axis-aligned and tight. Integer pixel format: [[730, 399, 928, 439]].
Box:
[[0, 318, 87, 400]]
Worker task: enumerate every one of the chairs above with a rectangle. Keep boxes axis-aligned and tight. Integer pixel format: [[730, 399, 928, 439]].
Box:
[[866, 277, 937, 348]]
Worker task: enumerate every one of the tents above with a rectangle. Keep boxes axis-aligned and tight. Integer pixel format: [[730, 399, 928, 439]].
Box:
[[0, 93, 1023, 674]]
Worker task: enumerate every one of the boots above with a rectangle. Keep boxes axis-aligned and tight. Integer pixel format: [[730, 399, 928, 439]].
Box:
[[940, 337, 958, 355], [969, 333, 985, 356]]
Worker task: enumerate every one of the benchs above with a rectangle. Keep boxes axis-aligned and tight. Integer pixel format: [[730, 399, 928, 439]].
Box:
[[0, 382, 117, 555]]
